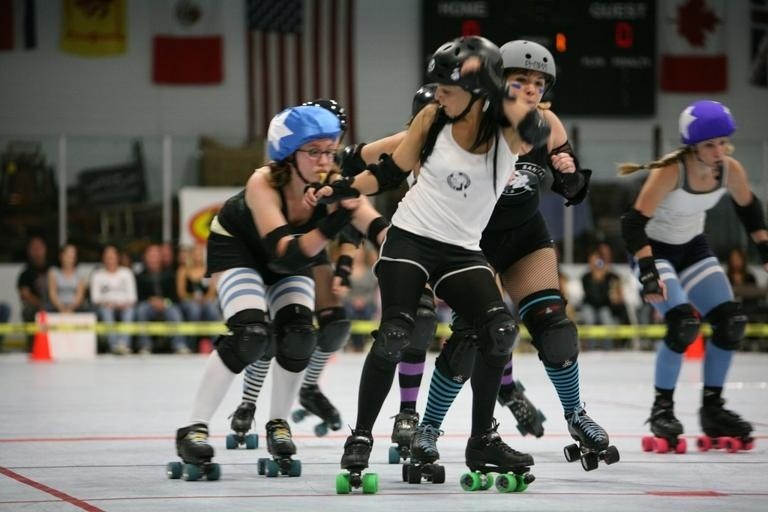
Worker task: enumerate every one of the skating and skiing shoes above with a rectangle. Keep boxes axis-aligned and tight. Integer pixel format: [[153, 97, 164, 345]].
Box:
[[642, 402, 687, 454], [227, 403, 259, 449], [402, 423, 446, 484], [166, 424, 221, 481], [498, 382, 547, 437], [293, 384, 341, 437], [389, 412, 419, 463], [564, 408, 620, 471], [257, 420, 302, 476], [696, 397, 754, 452], [460, 423, 534, 492], [337, 428, 377, 495]]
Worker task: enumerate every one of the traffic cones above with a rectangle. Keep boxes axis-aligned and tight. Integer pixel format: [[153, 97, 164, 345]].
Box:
[[685, 329, 707, 360], [27, 309, 54, 363]]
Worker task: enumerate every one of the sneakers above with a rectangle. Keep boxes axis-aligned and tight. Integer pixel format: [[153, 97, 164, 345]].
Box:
[[110, 340, 212, 355]]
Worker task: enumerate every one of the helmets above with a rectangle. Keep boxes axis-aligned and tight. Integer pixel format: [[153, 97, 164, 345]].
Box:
[[499, 40, 556, 96], [428, 35, 504, 92], [301, 98, 348, 143], [679, 100, 735, 144], [267, 106, 341, 162], [412, 82, 439, 119]]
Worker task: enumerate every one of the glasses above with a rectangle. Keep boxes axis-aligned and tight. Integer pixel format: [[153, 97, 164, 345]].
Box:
[[298, 147, 337, 158]]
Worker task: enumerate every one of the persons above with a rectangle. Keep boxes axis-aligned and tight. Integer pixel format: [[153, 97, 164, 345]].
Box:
[[299, 36, 558, 471], [153, 0, 223, 39], [230, 96, 413, 433], [622, 98, 766, 441], [176, 106, 394, 463], [409, 39, 610, 462], [580, 250, 662, 350], [663, 1, 727, 58], [338, 241, 380, 351], [15, 236, 223, 355], [727, 248, 761, 323], [391, 81, 543, 447]]
